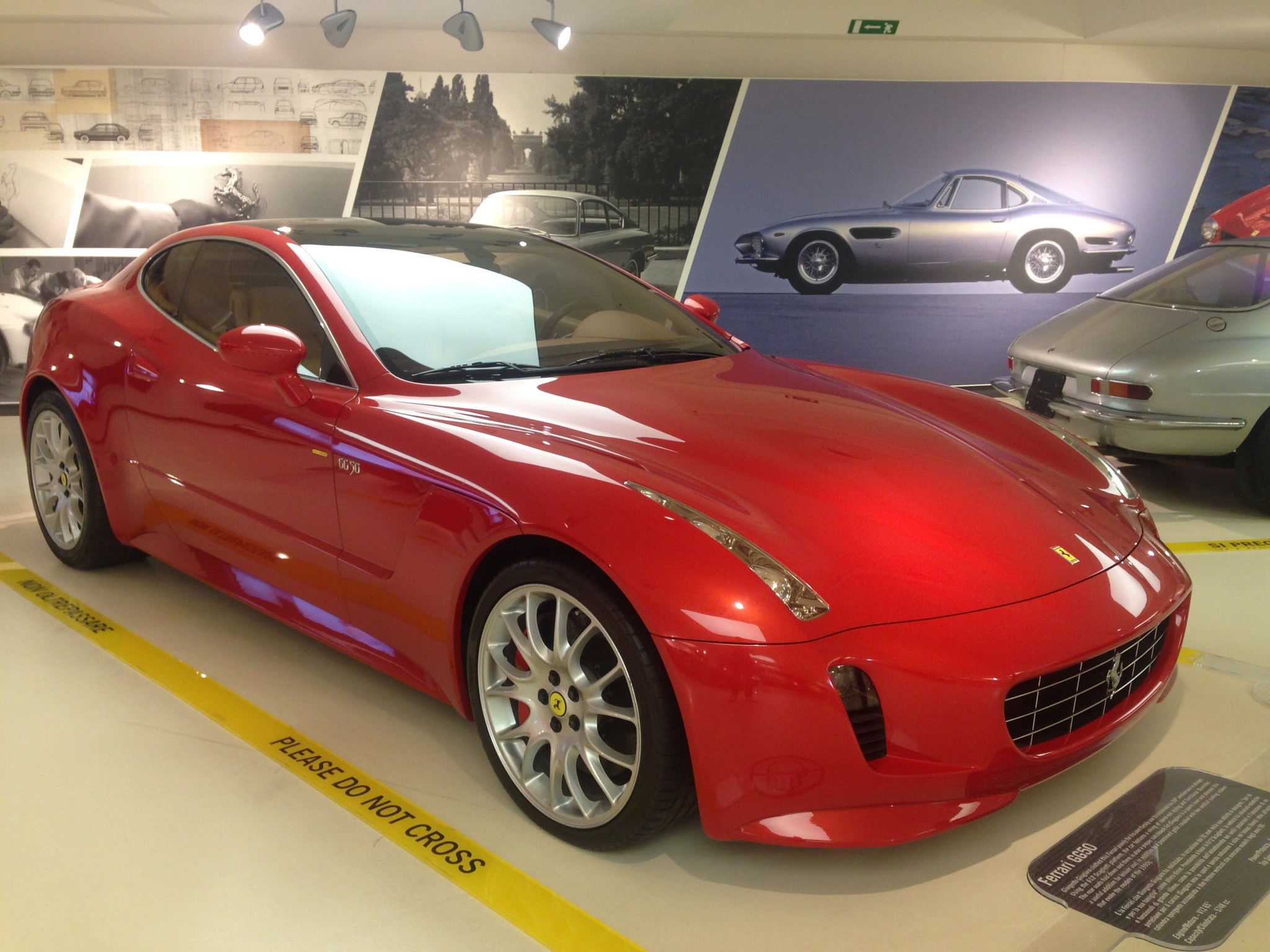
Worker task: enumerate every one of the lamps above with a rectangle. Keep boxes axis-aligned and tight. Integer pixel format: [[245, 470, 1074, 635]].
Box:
[[239, 1, 285, 46], [320, 0, 358, 48], [442, 0, 484, 52], [531, 0, 571, 51]]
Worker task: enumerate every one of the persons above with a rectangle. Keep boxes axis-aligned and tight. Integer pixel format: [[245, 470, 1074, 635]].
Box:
[[10, 259, 87, 299]]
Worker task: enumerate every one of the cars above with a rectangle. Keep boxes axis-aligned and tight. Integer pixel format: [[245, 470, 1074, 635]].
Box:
[[0, 292, 46, 376]]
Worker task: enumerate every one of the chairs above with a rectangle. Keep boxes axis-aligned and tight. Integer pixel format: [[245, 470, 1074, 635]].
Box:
[[235, 289, 323, 374]]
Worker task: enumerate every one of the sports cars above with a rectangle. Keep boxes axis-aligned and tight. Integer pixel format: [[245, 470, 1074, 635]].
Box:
[[737, 167, 1138, 296], [423, 191, 659, 311], [19, 216, 1194, 855], [991, 236, 1270, 518]]
